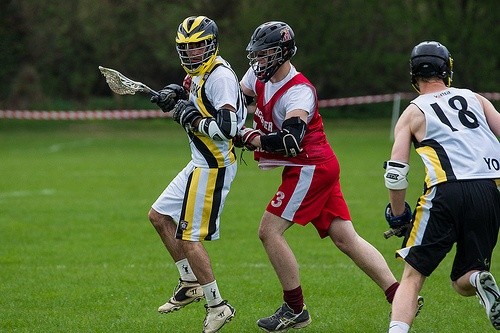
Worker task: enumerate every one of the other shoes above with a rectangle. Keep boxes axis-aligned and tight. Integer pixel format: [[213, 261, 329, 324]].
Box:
[[475, 270, 500, 331]]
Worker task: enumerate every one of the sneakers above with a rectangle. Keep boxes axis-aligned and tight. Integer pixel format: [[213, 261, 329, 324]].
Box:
[[158, 277, 205, 314], [202, 300, 237, 333], [257, 302, 312, 333], [415, 296, 424, 317]]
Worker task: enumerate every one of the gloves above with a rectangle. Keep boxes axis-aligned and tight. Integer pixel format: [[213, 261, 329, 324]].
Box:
[[172, 100, 204, 127], [232, 126, 265, 152], [151, 84, 187, 113], [385, 201, 413, 237]]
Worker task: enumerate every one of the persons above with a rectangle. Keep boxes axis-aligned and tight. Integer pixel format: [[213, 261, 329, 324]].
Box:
[[148, 16, 248, 333], [385, 41, 500, 333], [233, 21, 424, 333]]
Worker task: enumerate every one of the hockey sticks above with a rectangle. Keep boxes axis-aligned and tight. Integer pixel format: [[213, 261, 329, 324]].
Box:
[[384, 223, 413, 240], [98, 66, 160, 98]]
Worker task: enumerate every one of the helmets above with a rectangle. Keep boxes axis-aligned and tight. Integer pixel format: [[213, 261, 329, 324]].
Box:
[[176, 16, 220, 76], [410, 41, 453, 93], [246, 21, 296, 83]]
